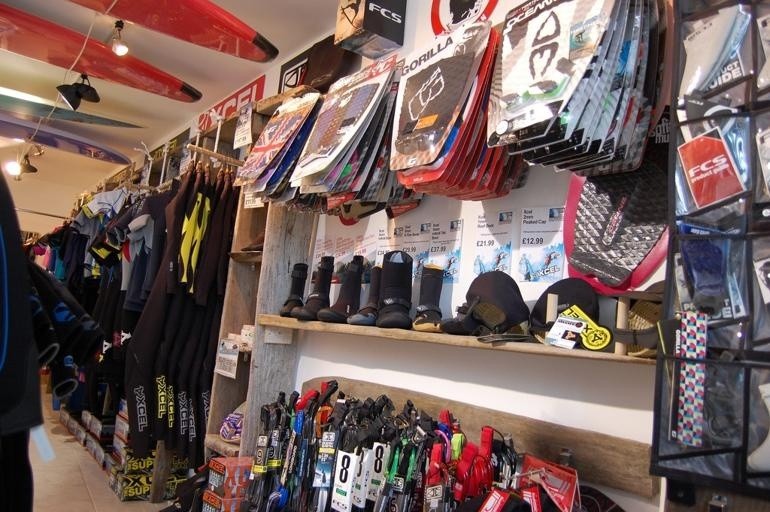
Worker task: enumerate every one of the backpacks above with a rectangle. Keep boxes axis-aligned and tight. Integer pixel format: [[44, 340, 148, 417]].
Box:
[[218, 400, 247, 444]]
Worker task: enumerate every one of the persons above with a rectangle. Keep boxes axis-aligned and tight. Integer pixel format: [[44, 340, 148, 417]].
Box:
[[0, 166, 45, 512]]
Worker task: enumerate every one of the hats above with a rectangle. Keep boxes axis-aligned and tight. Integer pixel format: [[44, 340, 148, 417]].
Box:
[[527, 276, 602, 345], [624, 278, 667, 359], [465, 267, 531, 335]]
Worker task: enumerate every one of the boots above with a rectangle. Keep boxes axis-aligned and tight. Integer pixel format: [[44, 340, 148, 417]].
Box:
[[289, 255, 336, 322], [279, 262, 310, 319], [412, 262, 445, 334], [375, 249, 414, 331], [316, 255, 365, 324], [346, 265, 383, 327]]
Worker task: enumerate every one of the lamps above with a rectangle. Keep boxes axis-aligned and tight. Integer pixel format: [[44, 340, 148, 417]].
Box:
[[3, 19, 142, 188]]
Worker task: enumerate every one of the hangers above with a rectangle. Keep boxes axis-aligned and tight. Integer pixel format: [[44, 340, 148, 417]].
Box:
[[66, 142, 245, 237], [22, 231, 47, 249]]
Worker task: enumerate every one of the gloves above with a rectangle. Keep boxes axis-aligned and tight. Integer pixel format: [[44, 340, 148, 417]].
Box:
[[680, 233, 731, 316]]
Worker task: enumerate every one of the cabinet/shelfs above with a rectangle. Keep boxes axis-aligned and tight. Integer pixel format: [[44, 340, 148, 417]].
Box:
[[204, 181, 318, 511]]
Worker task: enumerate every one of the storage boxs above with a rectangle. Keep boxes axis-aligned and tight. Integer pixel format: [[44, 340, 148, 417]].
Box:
[[60, 387, 189, 500], [202, 491, 243, 510], [208, 455, 256, 495]]
